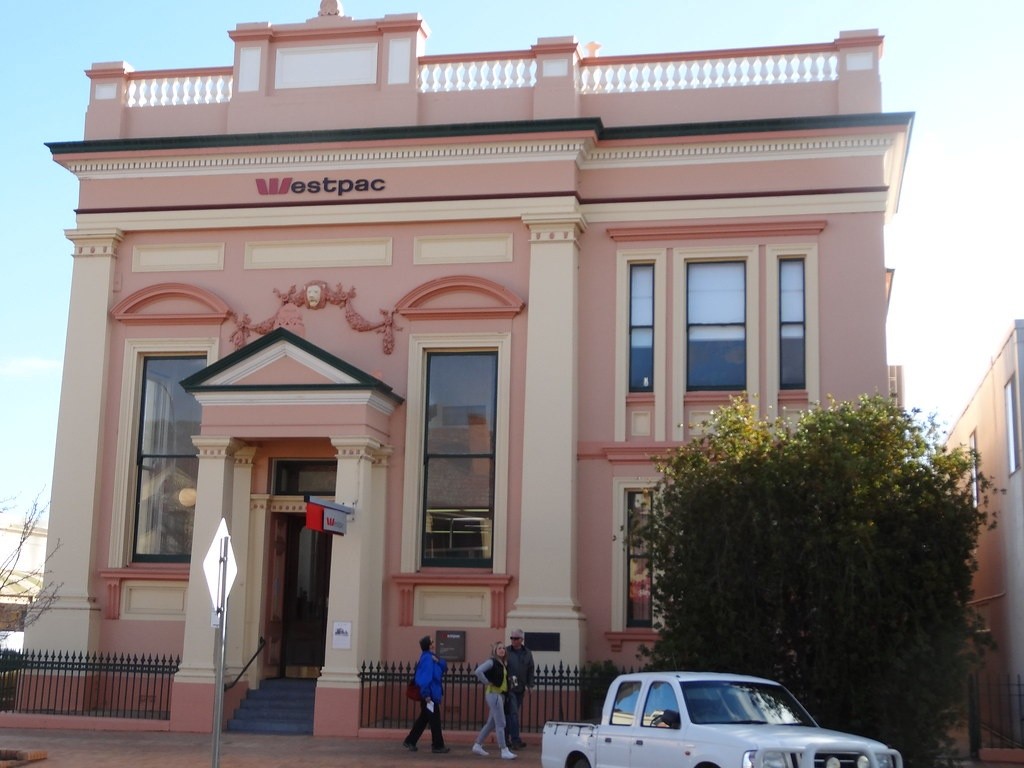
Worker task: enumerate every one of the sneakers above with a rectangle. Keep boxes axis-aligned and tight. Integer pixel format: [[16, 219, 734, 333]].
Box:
[[472, 742, 489, 756], [501, 747, 517, 759]]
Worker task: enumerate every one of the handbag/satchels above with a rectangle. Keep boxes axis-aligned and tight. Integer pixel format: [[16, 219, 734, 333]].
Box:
[[407, 683, 422, 701], [508, 674, 525, 692]]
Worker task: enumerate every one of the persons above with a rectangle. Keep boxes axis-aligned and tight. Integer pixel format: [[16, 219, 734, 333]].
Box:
[[501, 628, 535, 750], [472, 641, 518, 759], [403, 636, 449, 753]]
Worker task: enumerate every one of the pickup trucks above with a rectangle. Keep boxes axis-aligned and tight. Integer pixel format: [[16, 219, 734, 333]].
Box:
[[540, 672, 904, 768]]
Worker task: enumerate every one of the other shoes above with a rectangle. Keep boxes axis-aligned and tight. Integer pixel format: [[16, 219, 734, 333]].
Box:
[[512, 738, 526, 748], [403, 740, 417, 751], [432, 746, 449, 753]]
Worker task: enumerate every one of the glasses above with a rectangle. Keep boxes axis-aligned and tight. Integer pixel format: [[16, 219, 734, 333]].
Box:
[[510, 636, 524, 640], [431, 641, 434, 644]]
